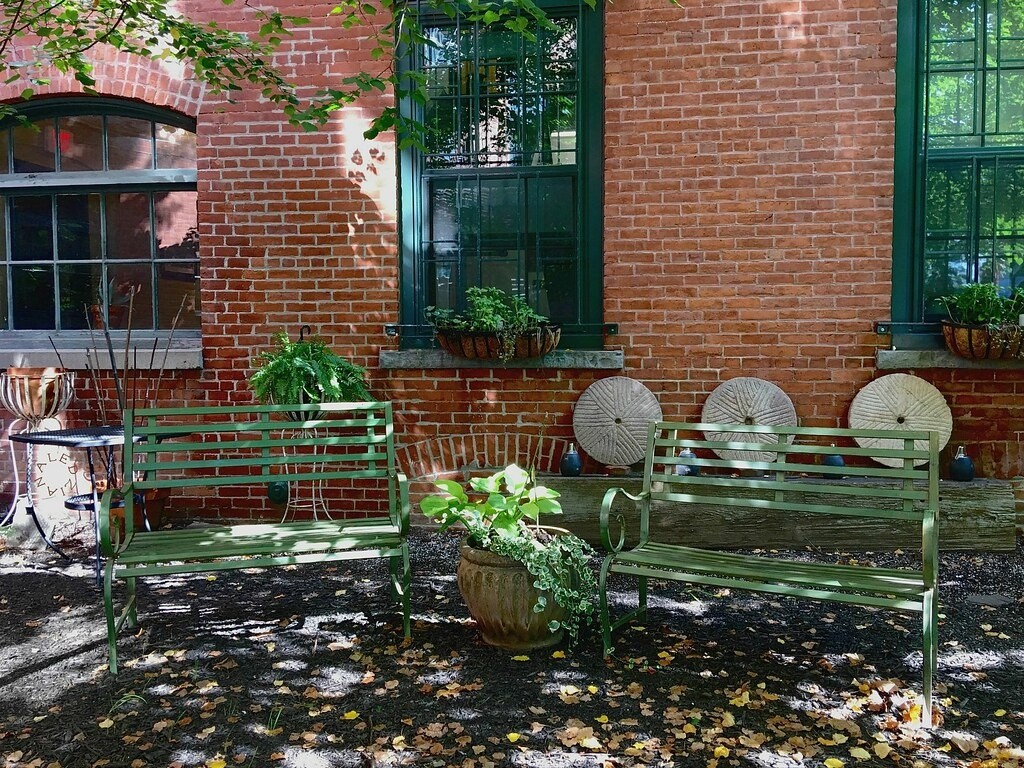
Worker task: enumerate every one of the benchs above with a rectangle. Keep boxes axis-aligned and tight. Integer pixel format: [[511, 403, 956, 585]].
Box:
[[102, 399, 411, 675], [598, 422, 941, 731]]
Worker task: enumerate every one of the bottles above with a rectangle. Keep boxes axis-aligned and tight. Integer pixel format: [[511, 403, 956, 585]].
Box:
[[676, 448, 697, 475], [822, 444, 844, 479], [560, 443, 582, 475], [950, 447, 974, 481]]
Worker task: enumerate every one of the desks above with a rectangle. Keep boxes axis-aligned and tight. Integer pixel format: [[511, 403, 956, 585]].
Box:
[[7, 427, 193, 589]]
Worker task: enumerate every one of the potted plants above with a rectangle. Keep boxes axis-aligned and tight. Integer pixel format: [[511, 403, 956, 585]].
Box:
[[92, 275, 143, 330], [248, 327, 379, 421], [933, 281, 1024, 361], [418, 462, 649, 670], [423, 286, 562, 370]]
